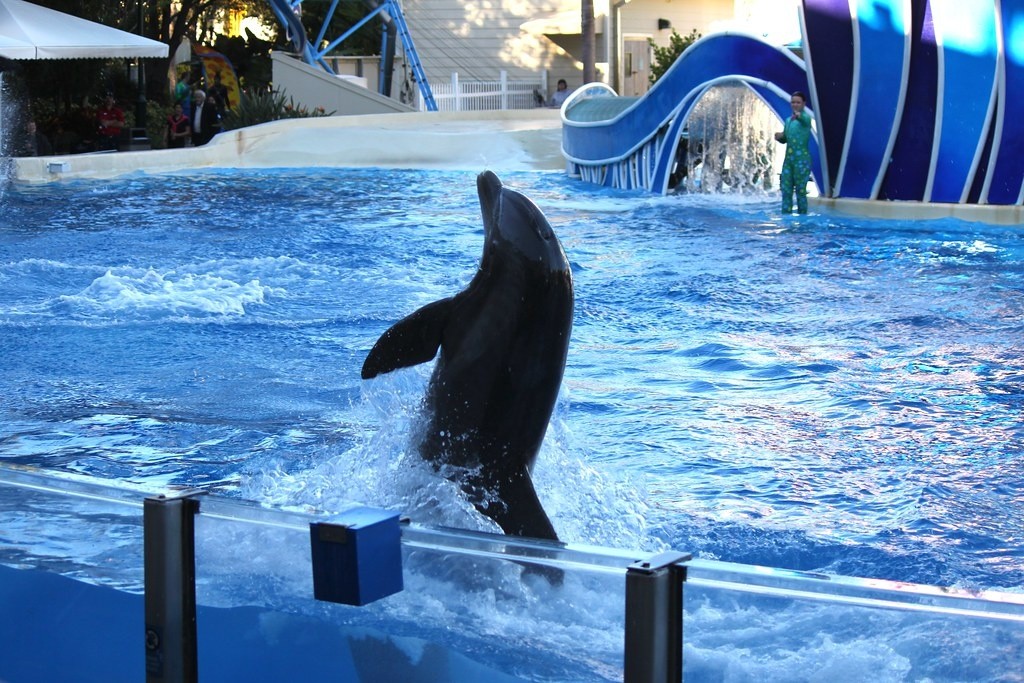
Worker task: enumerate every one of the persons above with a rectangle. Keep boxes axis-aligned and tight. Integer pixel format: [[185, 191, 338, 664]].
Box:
[[550, 80, 574, 107], [175, 72, 193, 115], [162, 103, 190, 149], [81, 90, 125, 151], [191, 90, 219, 148], [14, 119, 53, 157], [207, 74, 231, 116], [774, 91, 812, 215]]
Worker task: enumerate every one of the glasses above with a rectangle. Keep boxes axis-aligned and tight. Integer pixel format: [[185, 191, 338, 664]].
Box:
[[104, 91, 113, 96]]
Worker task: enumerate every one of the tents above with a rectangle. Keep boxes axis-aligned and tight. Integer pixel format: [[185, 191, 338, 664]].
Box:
[[0, 0, 169, 60]]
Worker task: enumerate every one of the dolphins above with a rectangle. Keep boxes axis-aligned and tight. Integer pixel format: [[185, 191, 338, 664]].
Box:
[[347, 171, 575, 683]]
